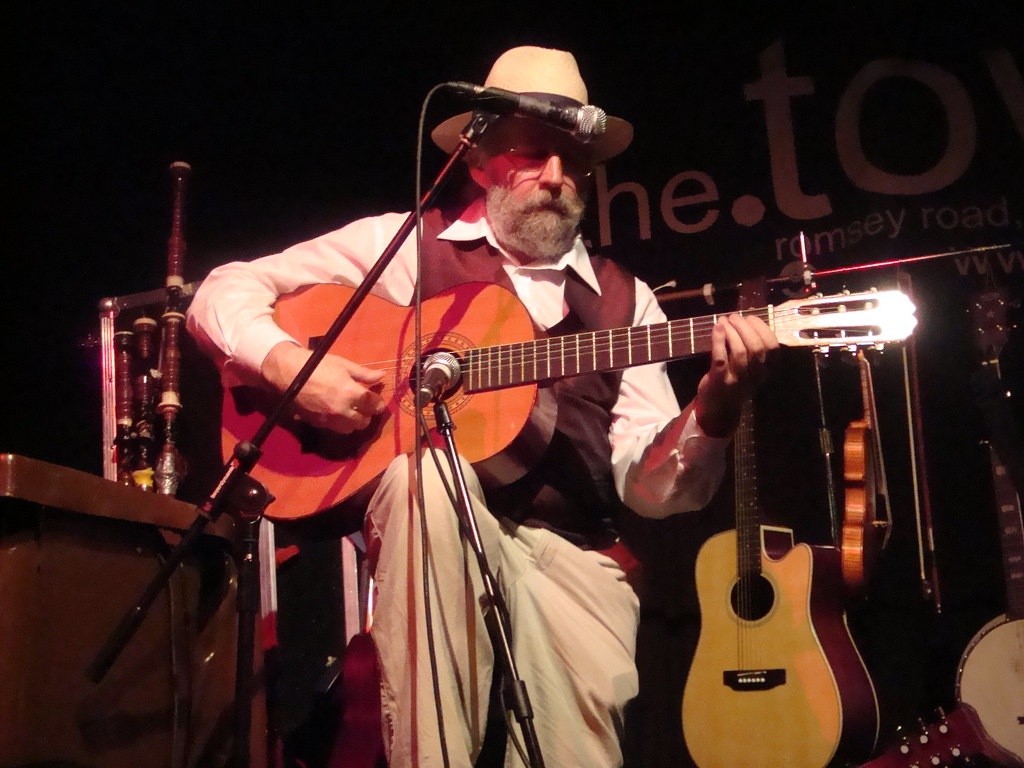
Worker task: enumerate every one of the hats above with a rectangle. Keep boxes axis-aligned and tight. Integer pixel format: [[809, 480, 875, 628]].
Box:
[[431, 46, 633, 166]]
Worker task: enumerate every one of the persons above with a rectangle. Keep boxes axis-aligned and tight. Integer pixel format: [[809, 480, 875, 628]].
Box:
[[187, 44, 783, 767]]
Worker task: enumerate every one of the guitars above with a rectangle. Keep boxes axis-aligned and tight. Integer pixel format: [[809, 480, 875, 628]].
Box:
[[954, 281, 1024, 768], [219, 279, 927, 541], [851, 705, 984, 768], [679, 272, 881, 768]]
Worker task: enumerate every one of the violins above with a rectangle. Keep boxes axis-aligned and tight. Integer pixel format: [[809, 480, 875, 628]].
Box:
[[840, 347, 896, 611]]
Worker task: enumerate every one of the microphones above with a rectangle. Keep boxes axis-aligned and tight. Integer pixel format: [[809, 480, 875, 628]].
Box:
[[414, 348, 461, 411], [446, 79, 608, 146]]
